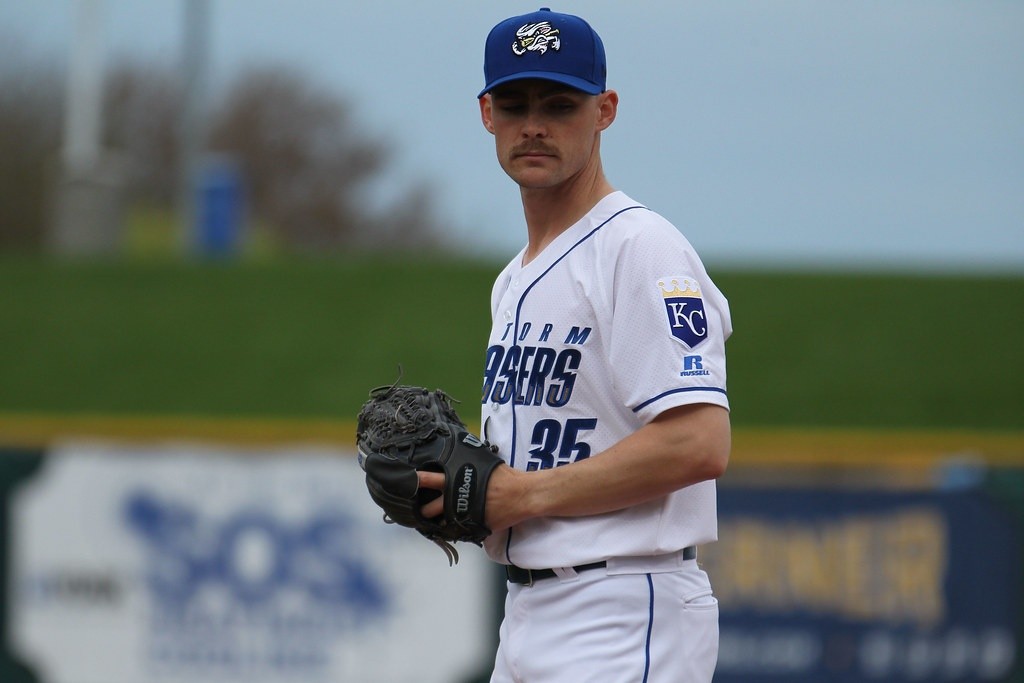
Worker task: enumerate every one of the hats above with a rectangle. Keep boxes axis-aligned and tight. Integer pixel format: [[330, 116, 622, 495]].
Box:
[[476, 7, 606, 100]]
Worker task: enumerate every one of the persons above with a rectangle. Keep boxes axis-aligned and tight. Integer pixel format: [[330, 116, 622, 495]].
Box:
[[416, 3, 734, 683]]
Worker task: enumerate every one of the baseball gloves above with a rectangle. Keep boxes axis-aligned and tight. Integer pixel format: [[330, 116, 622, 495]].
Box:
[[355, 362, 505, 565]]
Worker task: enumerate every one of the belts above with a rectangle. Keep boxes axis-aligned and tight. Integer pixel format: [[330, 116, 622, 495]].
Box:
[[505, 561, 608, 587]]
